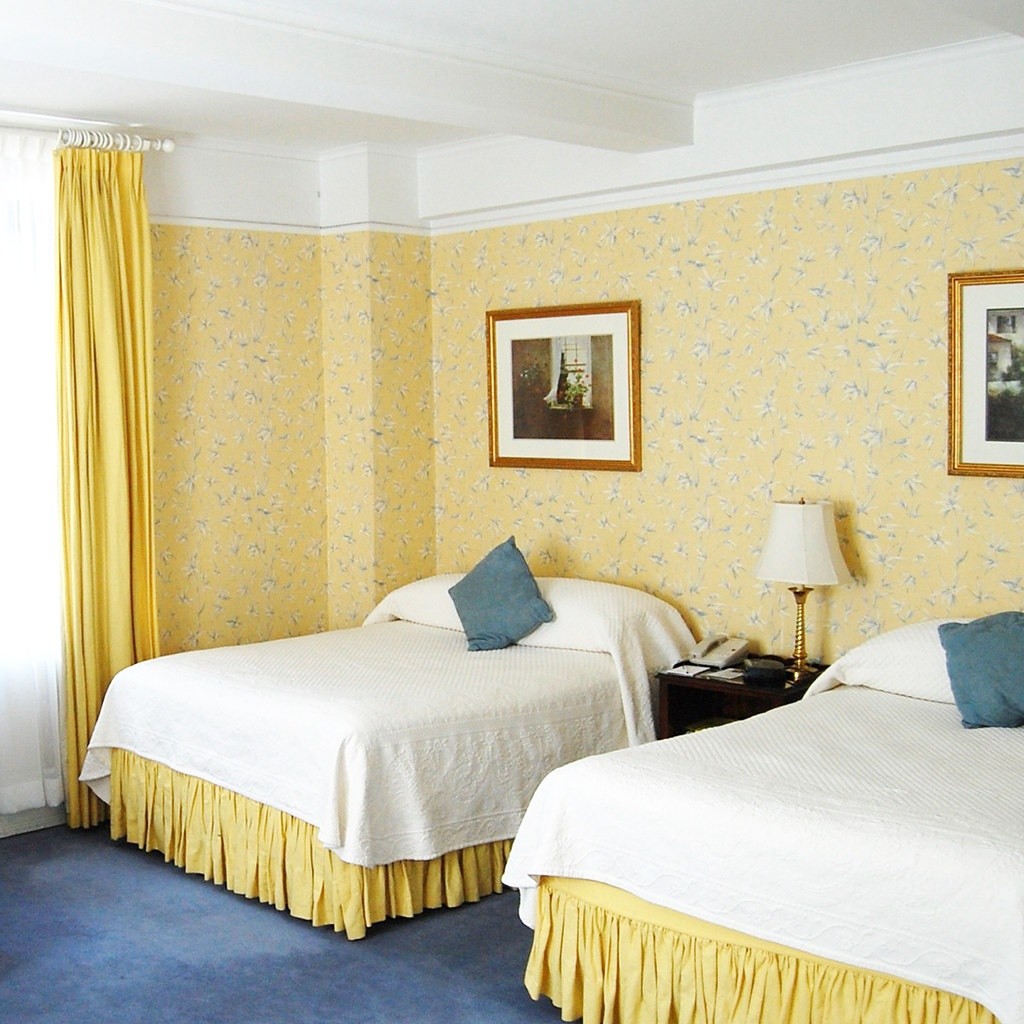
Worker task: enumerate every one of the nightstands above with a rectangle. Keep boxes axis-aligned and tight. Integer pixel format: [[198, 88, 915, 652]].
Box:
[[655, 655, 832, 740]]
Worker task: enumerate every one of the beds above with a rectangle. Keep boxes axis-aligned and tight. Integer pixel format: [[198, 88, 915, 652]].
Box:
[[501, 619, 1024, 1024], [78, 575, 696, 941]]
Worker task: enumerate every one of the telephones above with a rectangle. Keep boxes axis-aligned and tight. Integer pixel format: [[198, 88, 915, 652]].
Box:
[[689, 633, 750, 669]]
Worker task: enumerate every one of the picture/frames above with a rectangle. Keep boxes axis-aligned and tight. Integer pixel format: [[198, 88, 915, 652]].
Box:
[[484, 300, 641, 474], [947, 270, 1024, 480]]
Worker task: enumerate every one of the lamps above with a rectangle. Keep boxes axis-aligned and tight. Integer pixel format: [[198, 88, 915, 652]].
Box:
[[753, 500, 852, 683]]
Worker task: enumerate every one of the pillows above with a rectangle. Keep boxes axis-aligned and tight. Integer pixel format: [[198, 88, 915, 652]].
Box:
[[448, 535, 553, 653], [939, 611, 1024, 729]]
